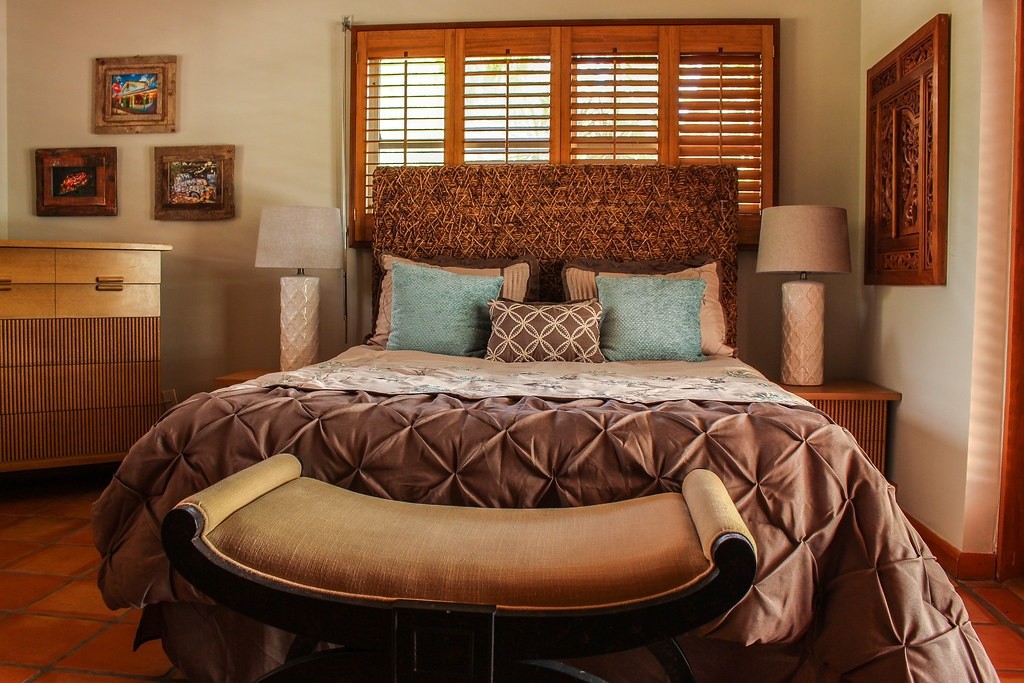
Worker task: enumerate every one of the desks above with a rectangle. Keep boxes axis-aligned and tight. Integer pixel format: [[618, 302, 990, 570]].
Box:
[[0, 241, 172, 478]]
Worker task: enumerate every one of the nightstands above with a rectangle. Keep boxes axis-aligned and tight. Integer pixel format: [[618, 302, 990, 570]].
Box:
[[767, 377, 902, 476], [214, 368, 280, 387]]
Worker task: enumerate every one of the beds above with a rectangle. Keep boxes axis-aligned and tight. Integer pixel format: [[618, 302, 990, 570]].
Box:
[[88, 166, 1002, 682]]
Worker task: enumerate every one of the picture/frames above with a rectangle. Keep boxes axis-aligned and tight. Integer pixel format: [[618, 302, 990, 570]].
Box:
[[153, 145, 236, 220], [35, 146, 118, 216], [91, 55, 175, 133]]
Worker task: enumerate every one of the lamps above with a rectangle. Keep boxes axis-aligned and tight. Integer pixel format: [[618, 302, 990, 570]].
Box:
[[255, 206, 345, 371], [755, 205, 852, 386]]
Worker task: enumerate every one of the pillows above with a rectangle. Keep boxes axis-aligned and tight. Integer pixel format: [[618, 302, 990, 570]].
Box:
[[362, 252, 540, 358], [483, 297, 607, 363], [561, 253, 739, 362]]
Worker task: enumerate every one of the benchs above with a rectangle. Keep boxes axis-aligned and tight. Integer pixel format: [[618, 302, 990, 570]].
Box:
[[160, 453, 758, 682]]
